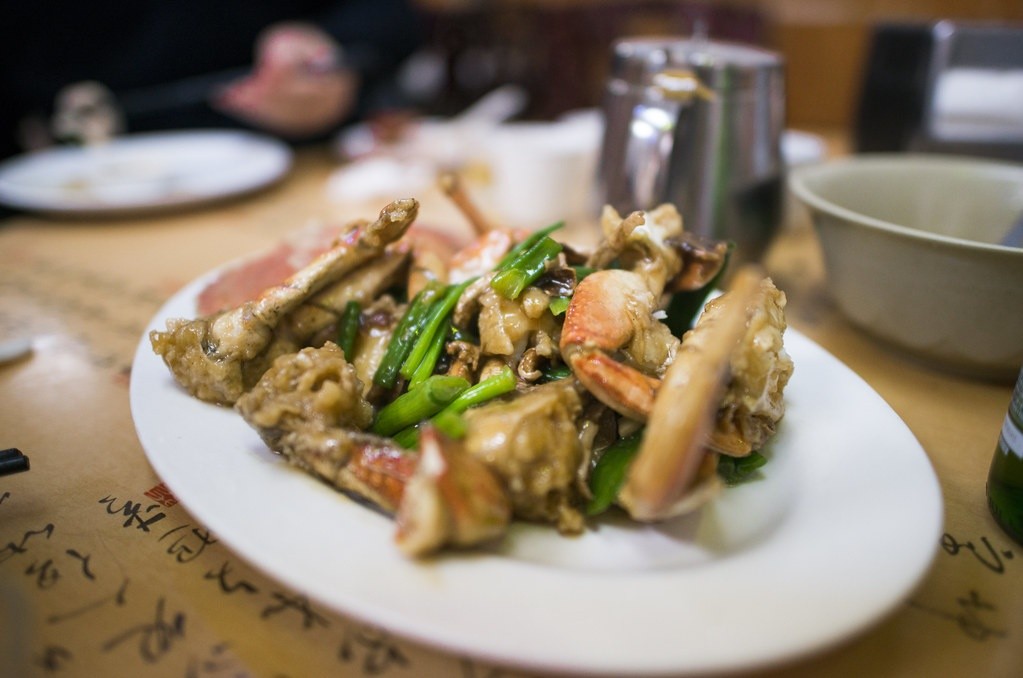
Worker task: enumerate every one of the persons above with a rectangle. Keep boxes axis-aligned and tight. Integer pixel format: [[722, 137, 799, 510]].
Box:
[[0, 0, 496, 155]]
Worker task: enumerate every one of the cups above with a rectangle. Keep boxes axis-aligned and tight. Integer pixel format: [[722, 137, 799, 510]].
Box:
[[588, 35, 791, 273]]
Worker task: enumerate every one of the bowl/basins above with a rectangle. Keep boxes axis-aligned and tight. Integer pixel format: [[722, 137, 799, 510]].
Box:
[[787, 149, 1023, 383]]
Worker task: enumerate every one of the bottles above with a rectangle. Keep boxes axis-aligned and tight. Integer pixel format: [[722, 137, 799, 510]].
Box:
[[986, 366, 1023, 548]]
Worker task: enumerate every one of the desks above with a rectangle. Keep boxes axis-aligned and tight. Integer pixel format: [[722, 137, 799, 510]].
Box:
[[2, 111, 1017, 676]]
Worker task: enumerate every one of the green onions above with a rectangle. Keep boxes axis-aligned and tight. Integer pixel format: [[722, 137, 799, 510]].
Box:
[[330, 226, 770, 528]]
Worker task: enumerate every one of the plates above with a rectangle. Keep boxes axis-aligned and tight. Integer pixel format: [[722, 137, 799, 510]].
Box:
[[127, 229, 945, 678], [0, 129, 293, 216]]
